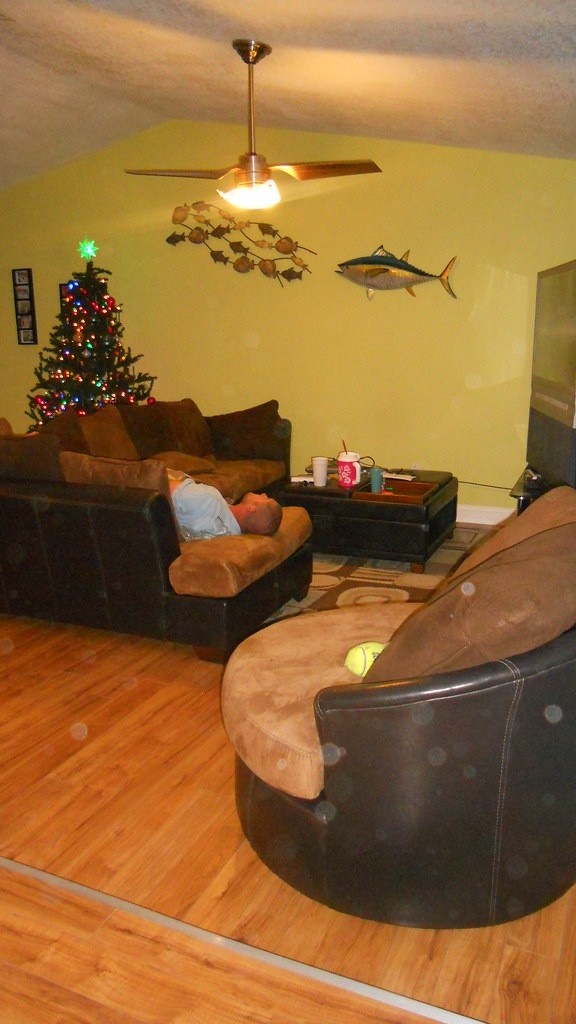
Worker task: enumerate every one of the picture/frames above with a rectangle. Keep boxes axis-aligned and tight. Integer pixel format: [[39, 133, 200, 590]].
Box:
[[60, 282, 69, 313], [12, 267, 37, 345]]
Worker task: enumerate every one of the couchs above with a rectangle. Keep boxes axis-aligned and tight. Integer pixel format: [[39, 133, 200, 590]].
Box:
[[0, 400, 314, 663], [221, 485, 576, 932]]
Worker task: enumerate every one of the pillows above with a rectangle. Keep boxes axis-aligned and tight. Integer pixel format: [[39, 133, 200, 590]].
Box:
[[115, 403, 174, 459], [38, 407, 89, 454], [1, 430, 64, 484], [363, 522, 576, 683], [203, 398, 285, 460], [61, 449, 186, 542], [80, 402, 141, 461], [446, 485, 576, 582], [153, 398, 214, 457], [147, 449, 215, 475]]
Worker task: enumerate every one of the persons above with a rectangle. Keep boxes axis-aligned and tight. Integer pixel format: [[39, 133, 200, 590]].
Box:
[[166, 469, 283, 540]]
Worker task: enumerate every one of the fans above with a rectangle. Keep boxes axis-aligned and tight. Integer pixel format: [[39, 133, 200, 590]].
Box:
[[117, 39, 385, 185]]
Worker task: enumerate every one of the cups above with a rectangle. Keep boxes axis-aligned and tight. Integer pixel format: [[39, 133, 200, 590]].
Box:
[[370, 469, 384, 494], [312, 457, 328, 487], [337, 451, 361, 486]]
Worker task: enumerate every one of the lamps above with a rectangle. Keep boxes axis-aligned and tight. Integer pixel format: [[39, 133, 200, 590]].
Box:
[[216, 170, 281, 211]]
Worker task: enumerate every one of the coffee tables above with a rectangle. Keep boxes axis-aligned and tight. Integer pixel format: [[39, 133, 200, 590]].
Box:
[[282, 467, 460, 574]]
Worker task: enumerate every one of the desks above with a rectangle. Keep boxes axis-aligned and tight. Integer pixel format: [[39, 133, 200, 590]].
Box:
[[510, 464, 555, 520]]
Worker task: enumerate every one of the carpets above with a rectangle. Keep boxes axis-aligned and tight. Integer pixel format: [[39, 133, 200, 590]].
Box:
[[257, 522, 495, 629]]
